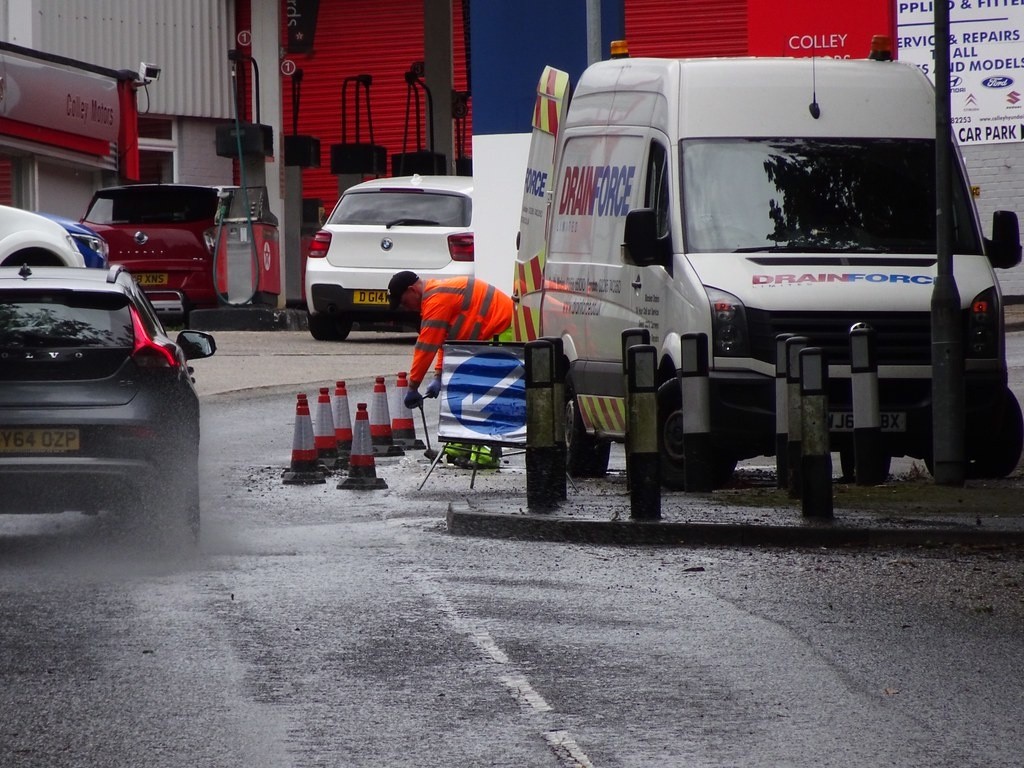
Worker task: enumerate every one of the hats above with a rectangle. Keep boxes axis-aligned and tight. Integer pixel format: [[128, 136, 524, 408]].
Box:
[[388, 271, 419, 311]]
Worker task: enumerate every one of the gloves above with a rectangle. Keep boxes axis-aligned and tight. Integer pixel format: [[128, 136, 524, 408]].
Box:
[[426, 378, 441, 399], [404, 391, 423, 409]]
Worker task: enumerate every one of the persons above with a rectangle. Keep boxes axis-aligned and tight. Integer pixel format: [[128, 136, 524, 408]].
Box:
[[386, 271, 515, 468]]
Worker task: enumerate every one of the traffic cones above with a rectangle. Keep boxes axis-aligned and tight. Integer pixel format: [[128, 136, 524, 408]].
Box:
[[280, 393, 326, 485], [333, 380, 353, 456], [369, 376, 406, 457], [313, 386, 347, 468], [336, 402, 389, 490], [391, 371, 426, 450]]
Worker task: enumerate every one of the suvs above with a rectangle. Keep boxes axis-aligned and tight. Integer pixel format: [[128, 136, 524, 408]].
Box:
[[0, 262, 218, 552]]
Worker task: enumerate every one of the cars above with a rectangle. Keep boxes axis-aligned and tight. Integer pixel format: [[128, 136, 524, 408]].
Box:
[[79, 183, 219, 326], [34, 212, 109, 268], [1, 204, 86, 269], [304, 176, 475, 342]]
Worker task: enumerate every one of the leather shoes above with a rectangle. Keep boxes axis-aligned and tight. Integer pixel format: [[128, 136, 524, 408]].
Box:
[[423, 448, 455, 462], [453, 457, 499, 469]]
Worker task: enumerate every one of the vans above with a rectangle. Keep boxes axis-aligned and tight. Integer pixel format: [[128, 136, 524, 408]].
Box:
[[511, 56, 1024, 495]]
